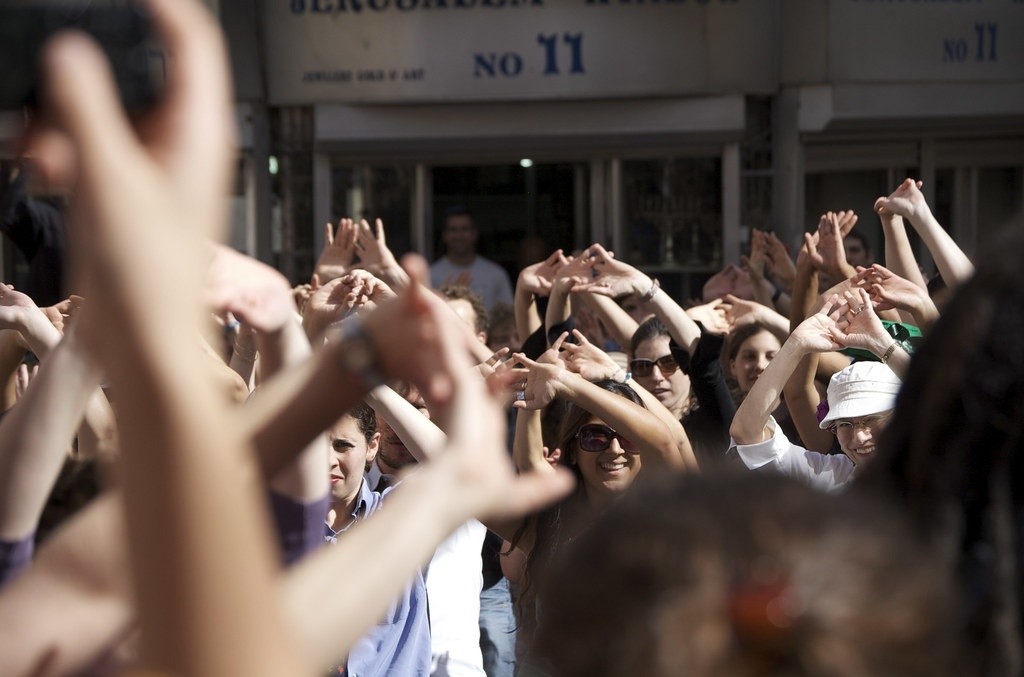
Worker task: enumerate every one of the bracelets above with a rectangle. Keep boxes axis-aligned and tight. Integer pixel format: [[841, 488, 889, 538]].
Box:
[[639, 279, 660, 303], [623, 373, 631, 384], [881, 343, 899, 364], [233, 338, 255, 362], [772, 289, 781, 304]]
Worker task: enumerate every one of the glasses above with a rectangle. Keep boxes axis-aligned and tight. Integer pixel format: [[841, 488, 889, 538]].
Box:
[[888, 323, 925, 356], [825, 409, 897, 435], [573, 423, 642, 454], [631, 353, 681, 378]]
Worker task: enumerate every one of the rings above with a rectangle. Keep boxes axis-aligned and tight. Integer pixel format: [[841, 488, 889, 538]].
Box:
[[517, 391, 525, 400], [855, 308, 863, 313], [522, 383, 525, 390]]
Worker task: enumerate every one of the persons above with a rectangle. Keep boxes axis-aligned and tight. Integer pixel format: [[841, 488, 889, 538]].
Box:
[[0, 0, 1024, 677]]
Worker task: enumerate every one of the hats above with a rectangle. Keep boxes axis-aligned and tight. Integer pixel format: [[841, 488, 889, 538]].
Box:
[[819, 360, 904, 429]]
